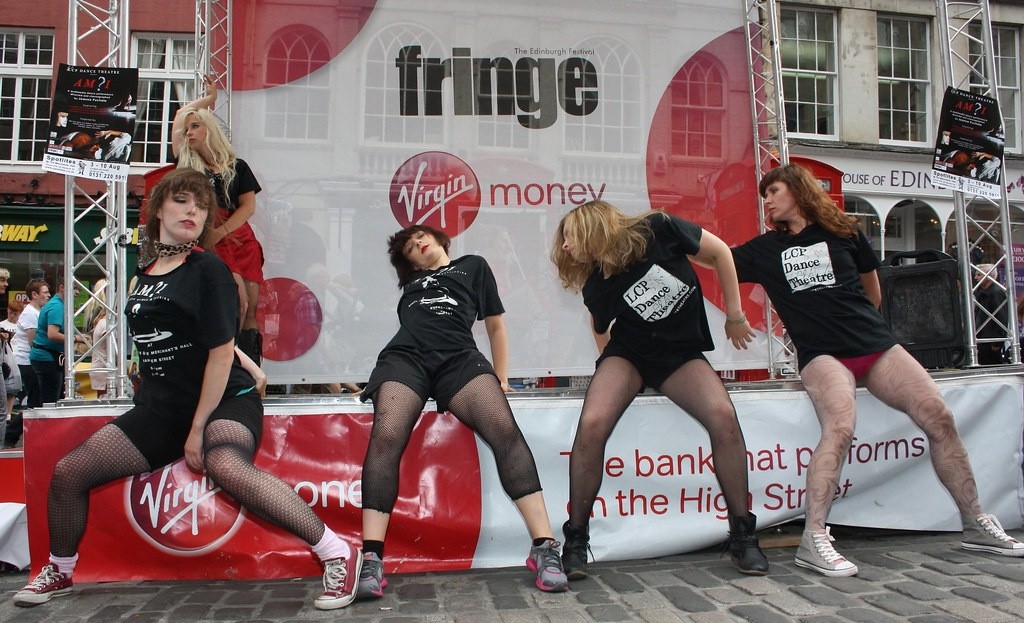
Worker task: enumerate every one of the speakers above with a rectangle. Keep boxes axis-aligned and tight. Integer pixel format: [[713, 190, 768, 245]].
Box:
[[878, 249, 963, 369]]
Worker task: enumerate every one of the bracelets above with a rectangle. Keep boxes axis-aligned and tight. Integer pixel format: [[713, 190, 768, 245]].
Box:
[[726, 311, 746, 324]]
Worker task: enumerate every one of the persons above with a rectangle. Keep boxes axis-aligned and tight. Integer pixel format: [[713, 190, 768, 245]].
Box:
[[54, 130, 131, 163], [357, 225, 568, 598], [172, 73, 265, 368], [13, 168, 364, 609], [0, 268, 117, 450], [89, 280, 111, 398], [941, 131, 1001, 185], [957, 264, 1024, 365], [550, 201, 770, 580], [290, 383, 368, 394], [685, 164, 1024, 578]]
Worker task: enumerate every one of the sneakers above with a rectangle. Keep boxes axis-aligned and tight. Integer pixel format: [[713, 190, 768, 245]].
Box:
[[796, 526, 858, 576], [14, 563, 73, 606], [316, 541, 363, 609], [561, 521, 595, 579], [357, 552, 387, 598], [726, 513, 768, 574], [962, 514, 1024, 557], [526, 540, 568, 591]]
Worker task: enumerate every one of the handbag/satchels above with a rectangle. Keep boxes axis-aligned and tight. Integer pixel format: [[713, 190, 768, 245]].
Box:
[[0, 339, 23, 393]]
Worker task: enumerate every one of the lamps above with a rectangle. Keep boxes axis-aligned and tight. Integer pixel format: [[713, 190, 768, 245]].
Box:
[[129, 190, 141, 205], [4, 193, 14, 203], [37, 195, 44, 204]]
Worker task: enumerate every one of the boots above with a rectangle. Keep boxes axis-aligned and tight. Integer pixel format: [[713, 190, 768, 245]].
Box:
[[238, 327, 263, 370]]
